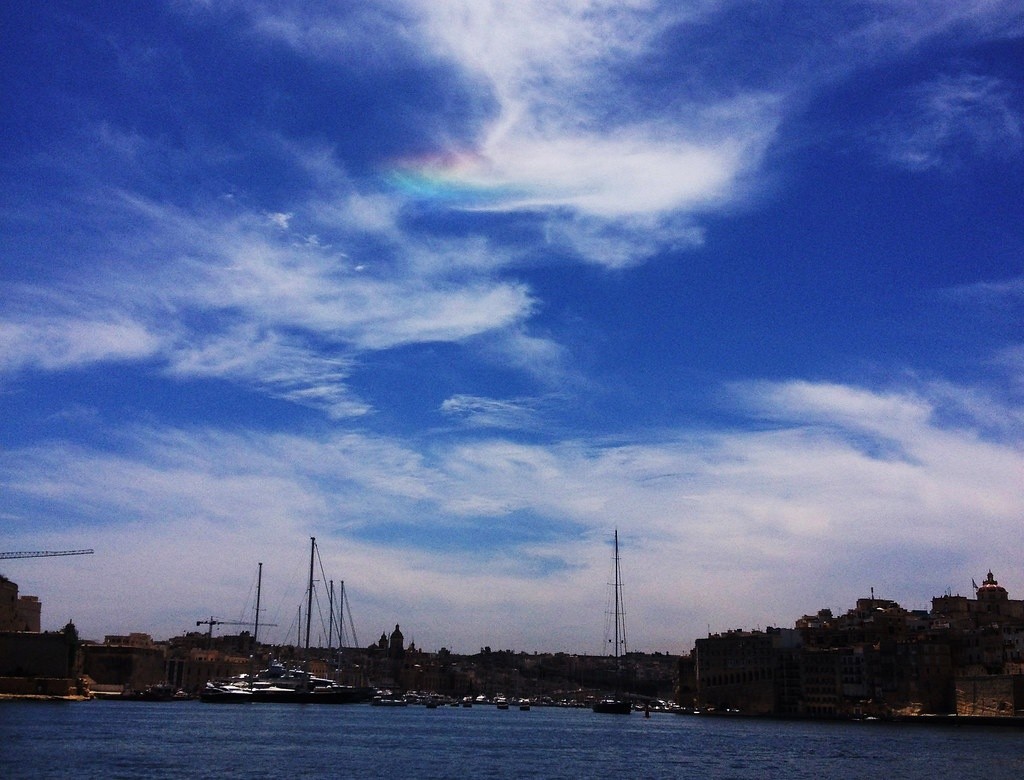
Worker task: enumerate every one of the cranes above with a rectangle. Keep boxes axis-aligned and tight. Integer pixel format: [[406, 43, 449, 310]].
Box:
[[197, 615, 278, 650], [0, 548, 95, 559]]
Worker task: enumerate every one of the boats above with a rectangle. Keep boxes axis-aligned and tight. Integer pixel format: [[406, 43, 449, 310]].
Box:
[[145, 679, 180, 700], [198, 533, 531, 711]]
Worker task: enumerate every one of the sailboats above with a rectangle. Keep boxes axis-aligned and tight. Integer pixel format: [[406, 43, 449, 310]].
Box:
[[589, 525, 636, 715]]
